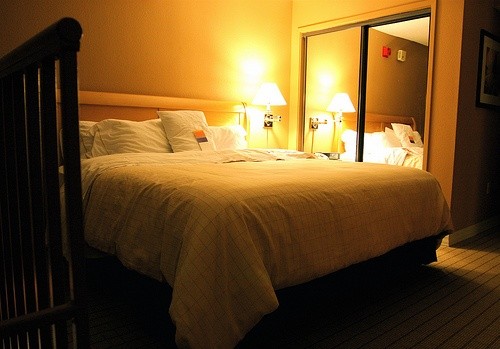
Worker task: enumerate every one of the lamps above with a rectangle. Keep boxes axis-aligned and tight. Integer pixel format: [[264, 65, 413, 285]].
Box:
[[253, 83, 287, 129], [309, 92, 356, 131]]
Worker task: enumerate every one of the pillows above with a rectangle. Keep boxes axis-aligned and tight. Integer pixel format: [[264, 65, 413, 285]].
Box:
[[342, 129, 390, 153], [88, 118, 173, 157], [391, 123, 418, 147], [385, 127, 402, 148], [60, 117, 165, 161], [413, 132, 423, 148], [209, 126, 248, 150], [157, 110, 217, 153]]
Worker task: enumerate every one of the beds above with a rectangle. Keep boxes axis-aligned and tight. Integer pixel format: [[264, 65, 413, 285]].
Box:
[[333, 112, 424, 171], [39, 90, 454, 349]]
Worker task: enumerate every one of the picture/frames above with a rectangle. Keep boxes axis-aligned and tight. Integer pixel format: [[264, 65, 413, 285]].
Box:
[[476, 29, 500, 110]]
